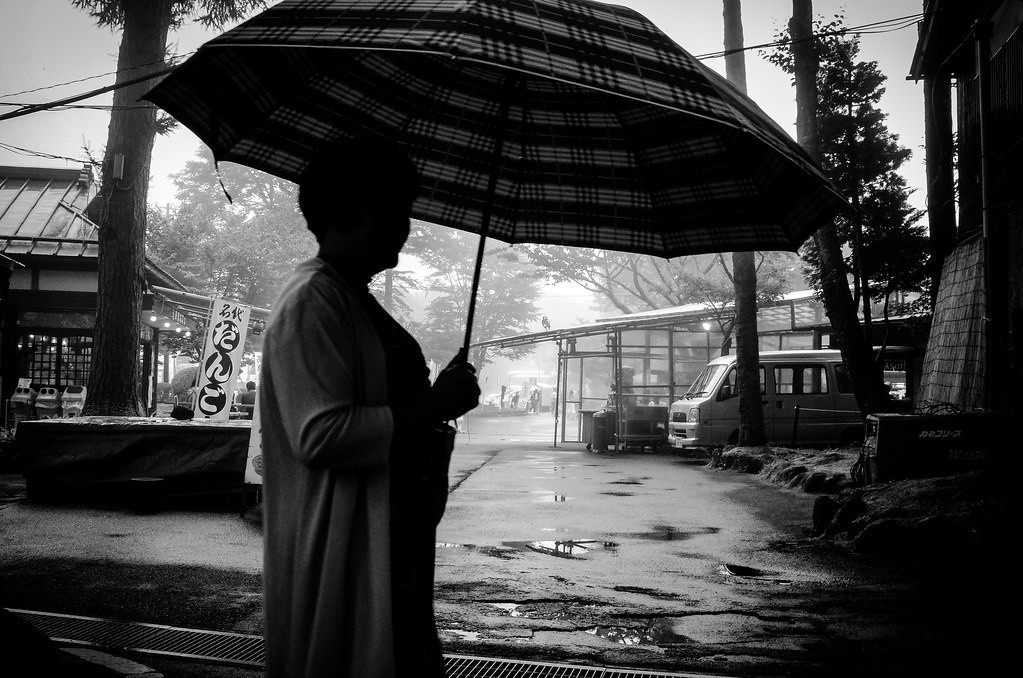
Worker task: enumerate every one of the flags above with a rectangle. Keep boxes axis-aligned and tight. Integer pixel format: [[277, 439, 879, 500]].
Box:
[[193, 300, 252, 420], [245, 387, 263, 484]]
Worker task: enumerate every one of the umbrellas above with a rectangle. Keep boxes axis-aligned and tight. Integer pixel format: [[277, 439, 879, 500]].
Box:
[[136, 1, 858, 364]]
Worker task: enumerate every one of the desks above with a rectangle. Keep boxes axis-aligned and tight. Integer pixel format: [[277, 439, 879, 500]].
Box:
[[579, 410, 615, 450]]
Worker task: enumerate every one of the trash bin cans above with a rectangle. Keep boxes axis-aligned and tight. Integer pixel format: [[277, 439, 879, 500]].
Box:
[[592, 409, 616, 451], [35, 387, 61, 419], [10, 387, 37, 437], [62, 386, 87, 418]]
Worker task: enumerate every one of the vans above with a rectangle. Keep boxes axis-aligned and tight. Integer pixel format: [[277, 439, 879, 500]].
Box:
[[872, 346, 910, 400], [668, 349, 867, 453]]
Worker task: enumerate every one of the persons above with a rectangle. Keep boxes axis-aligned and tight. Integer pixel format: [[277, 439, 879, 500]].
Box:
[[258, 135, 481, 678], [640, 390, 666, 407], [229, 381, 257, 419], [511, 388, 576, 416]]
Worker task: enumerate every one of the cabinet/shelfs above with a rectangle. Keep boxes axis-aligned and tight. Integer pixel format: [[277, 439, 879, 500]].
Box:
[[615, 407, 668, 450]]
